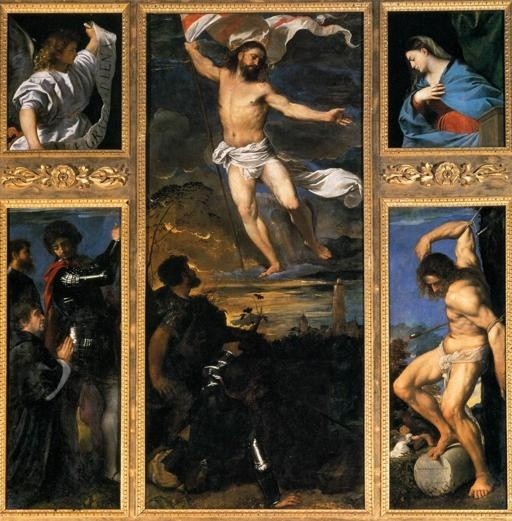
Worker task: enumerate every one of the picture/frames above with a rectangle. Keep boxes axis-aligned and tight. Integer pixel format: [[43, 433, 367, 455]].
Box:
[[379, 2, 510, 157], [381, 195, 511, 520], [3, 200, 130, 518], [1, 2, 131, 156], [135, 2, 373, 520]]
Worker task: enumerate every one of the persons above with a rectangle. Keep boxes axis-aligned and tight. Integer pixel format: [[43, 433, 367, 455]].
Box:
[[393, 221, 506, 498], [7, 220, 121, 508], [333, 278, 346, 321], [145, 255, 202, 489], [399, 36, 503, 148], [185, 40, 352, 277], [12, 23, 98, 150]]
